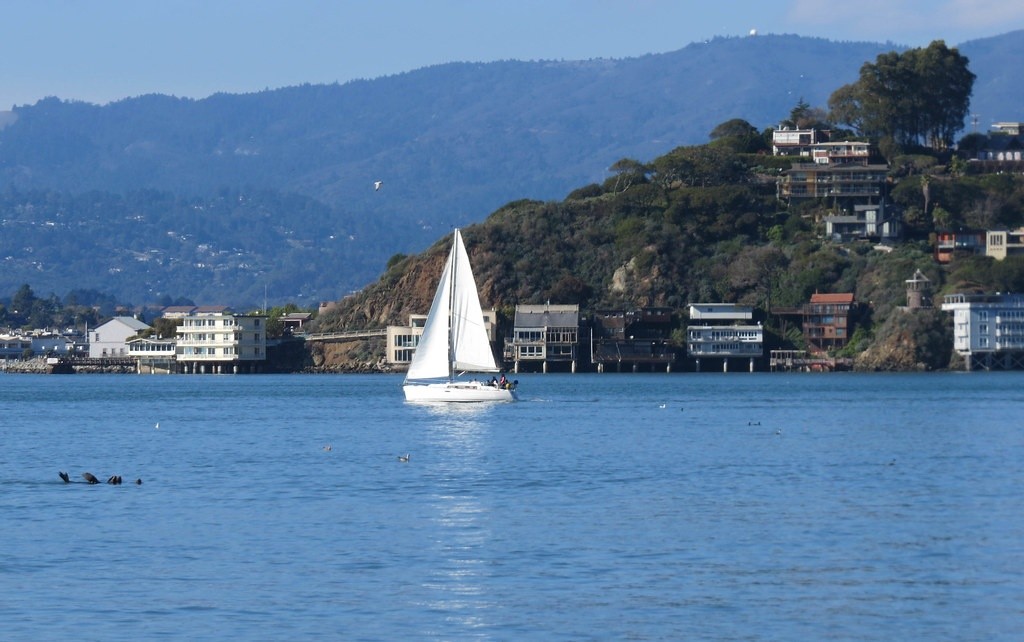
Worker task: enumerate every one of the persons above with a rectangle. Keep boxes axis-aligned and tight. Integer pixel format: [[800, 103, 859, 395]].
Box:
[[491, 373, 506, 389]]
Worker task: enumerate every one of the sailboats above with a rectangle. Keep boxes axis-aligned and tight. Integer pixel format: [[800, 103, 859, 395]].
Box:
[[402, 228, 518, 402]]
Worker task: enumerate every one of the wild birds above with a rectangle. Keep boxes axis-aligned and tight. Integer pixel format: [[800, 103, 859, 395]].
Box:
[[680, 407, 684, 412], [775, 427, 782, 436], [57, 471, 142, 485], [396, 452, 411, 465], [748, 421, 762, 426], [322, 445, 332, 452], [889, 457, 897, 466], [154, 421, 160, 429], [659, 403, 665, 409], [374, 179, 384, 191]]
